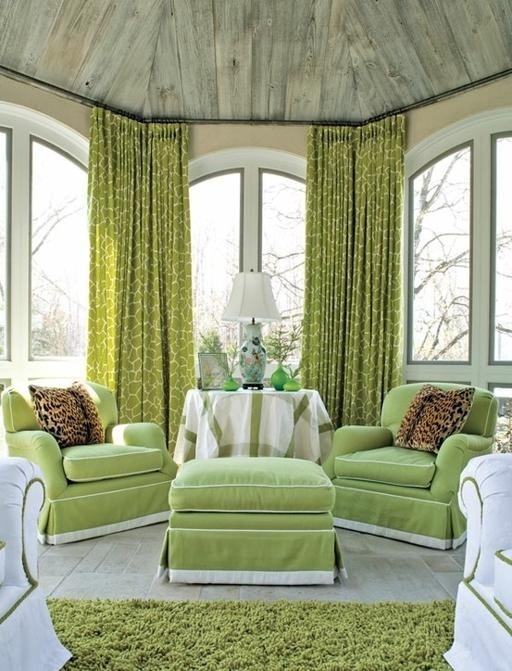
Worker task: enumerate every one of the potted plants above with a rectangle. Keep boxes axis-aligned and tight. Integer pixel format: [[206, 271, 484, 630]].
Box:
[[284, 363, 305, 392], [266, 319, 308, 389], [197, 330, 242, 390]]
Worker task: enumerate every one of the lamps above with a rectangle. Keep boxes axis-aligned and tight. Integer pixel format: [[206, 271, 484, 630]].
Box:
[[222, 271, 282, 390]]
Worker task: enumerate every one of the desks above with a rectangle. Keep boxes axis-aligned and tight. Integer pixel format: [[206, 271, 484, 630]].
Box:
[[182, 387, 318, 462]]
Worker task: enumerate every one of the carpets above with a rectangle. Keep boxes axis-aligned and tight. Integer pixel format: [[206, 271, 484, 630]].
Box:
[[45, 597, 455, 671]]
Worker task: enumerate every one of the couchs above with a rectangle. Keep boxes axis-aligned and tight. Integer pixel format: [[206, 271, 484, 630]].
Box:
[[164, 458, 337, 578], [0, 380, 176, 545], [328, 381, 498, 550], [1, 460, 66, 671], [454, 452, 512, 669]]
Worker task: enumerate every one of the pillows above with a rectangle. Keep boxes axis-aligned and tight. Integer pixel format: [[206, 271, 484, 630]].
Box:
[[28, 381, 105, 448], [394, 383, 474, 451]]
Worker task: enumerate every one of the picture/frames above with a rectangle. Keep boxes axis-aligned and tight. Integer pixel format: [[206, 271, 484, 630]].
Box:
[[197, 353, 231, 389]]
